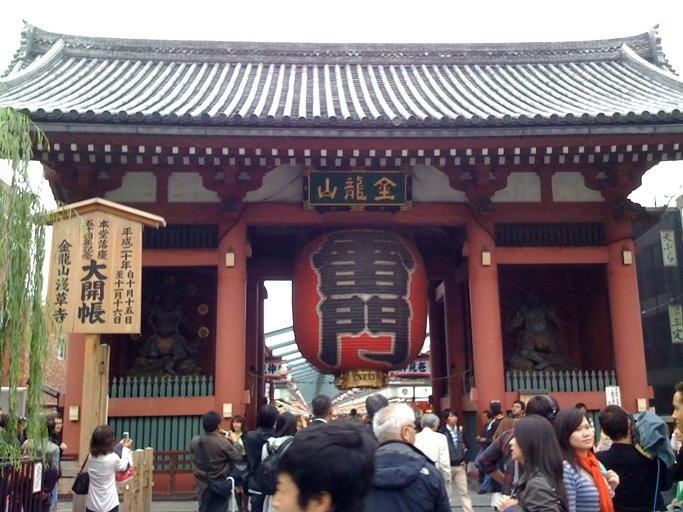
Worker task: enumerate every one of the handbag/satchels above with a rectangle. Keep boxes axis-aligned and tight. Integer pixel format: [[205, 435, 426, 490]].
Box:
[[464, 448, 470, 463], [72, 472, 89, 494], [116, 452, 136, 483], [240, 466, 250, 494], [209, 468, 241, 501]]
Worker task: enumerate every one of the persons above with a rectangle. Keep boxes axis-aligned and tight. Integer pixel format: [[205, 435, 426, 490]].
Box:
[[84, 425, 134, 512], [270, 421, 376, 512], [189, 382, 683, 512], [0, 408, 67, 512]]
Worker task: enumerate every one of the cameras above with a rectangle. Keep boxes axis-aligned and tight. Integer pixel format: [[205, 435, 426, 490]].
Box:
[[222, 431, 229, 441]]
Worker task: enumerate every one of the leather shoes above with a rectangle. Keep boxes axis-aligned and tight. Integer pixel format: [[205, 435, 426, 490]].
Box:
[[478, 490, 487, 494]]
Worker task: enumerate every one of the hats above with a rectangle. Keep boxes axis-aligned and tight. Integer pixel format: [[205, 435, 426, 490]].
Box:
[[490, 399, 502, 412]]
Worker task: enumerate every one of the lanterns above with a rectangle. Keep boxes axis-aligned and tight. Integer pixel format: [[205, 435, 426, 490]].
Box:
[[291, 228, 430, 389]]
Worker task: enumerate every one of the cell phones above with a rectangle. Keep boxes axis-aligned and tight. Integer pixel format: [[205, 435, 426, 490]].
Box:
[[123, 432, 130, 439]]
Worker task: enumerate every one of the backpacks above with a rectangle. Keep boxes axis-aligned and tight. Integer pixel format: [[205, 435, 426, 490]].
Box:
[[257, 437, 293, 495]]
